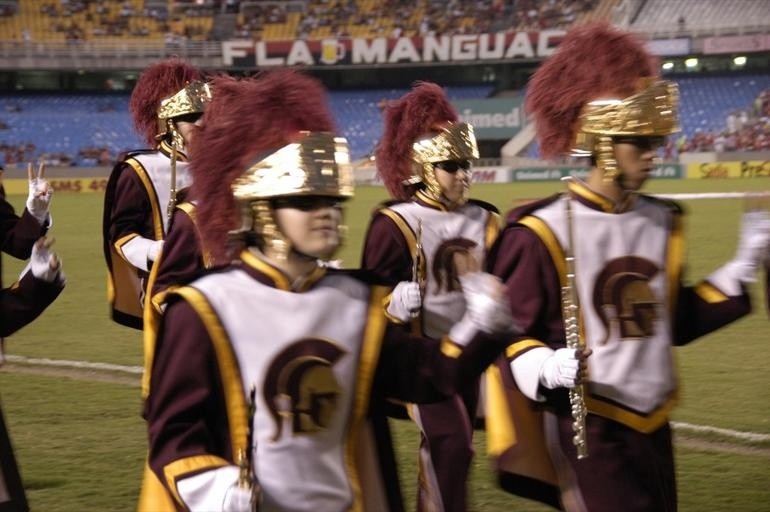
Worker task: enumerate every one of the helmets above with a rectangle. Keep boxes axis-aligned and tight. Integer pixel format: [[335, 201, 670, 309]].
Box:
[[567, 80, 680, 189], [404, 121, 480, 203], [229, 129, 349, 263], [157, 81, 213, 154]]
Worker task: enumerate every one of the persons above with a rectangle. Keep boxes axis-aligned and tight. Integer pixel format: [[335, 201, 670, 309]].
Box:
[[664, 86, 769, 164], [136, 67, 512, 512], [490, 23, 769, 512], [1, 235, 69, 511], [100, 55, 218, 333], [140, 166, 221, 401], [1, 1, 591, 46], [1, 161, 60, 271], [0, 73, 121, 169], [0, 260, 32, 289], [361, 82, 506, 512]]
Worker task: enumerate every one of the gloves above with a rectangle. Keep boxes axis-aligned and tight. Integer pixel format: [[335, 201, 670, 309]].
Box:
[[460, 270, 516, 333], [29, 239, 64, 282], [540, 346, 585, 390], [221, 483, 255, 512], [725, 205, 770, 285], [386, 279, 422, 319], [147, 240, 164, 262], [23, 176, 54, 226]]
